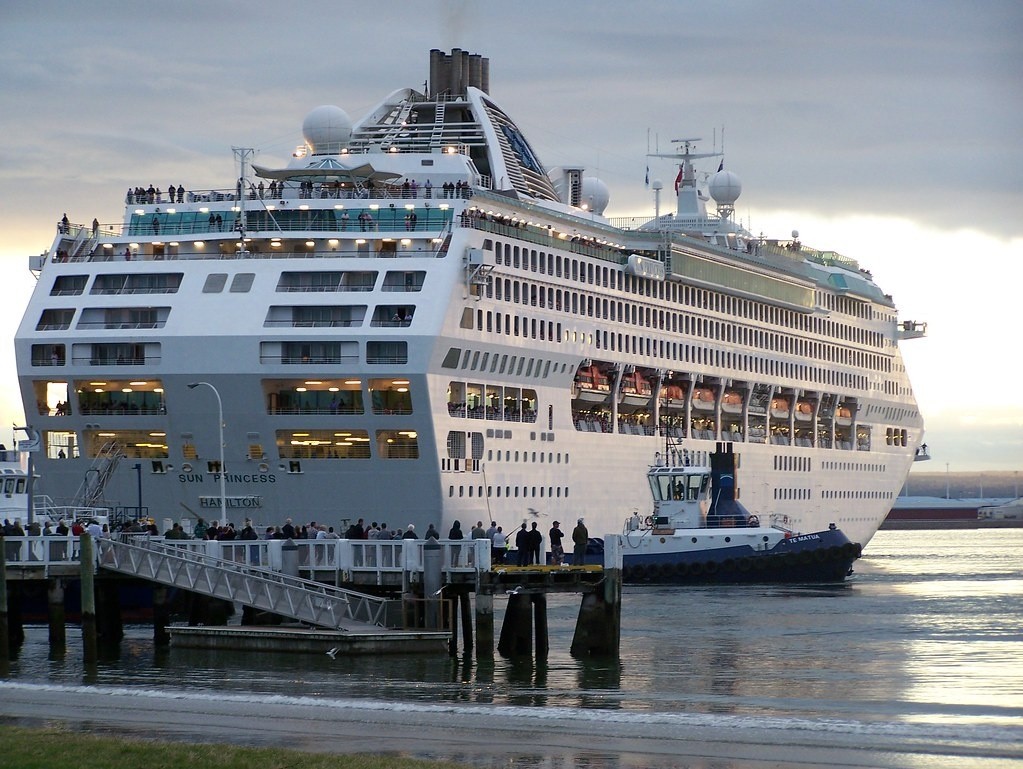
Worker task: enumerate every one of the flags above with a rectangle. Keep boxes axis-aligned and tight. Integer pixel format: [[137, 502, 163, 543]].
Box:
[[674, 168, 683, 195], [718, 159, 723, 172], [646, 166, 650, 184]]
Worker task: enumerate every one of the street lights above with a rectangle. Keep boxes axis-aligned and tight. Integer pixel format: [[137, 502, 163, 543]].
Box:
[[186, 381, 227, 528], [1014, 471, 1018, 499], [946, 463, 949, 500], [980, 473, 984, 498]]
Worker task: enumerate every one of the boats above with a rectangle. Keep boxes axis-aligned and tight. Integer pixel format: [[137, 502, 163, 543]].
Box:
[[621, 372, 652, 396], [574, 366, 609, 390], [504, 440, 862, 585], [0, 444, 109, 535]]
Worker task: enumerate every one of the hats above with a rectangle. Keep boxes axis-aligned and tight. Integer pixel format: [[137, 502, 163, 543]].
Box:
[[577, 518, 584, 522], [364, 212, 369, 215], [45, 522, 53, 526], [149, 184, 153, 186], [246, 517, 252, 521], [552, 520, 560, 524]]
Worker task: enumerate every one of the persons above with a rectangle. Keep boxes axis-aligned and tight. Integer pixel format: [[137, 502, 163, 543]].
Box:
[[405, 313, 413, 326], [666, 480, 684, 501], [41, 177, 617, 262], [54, 393, 846, 459], [0, 517, 588, 566], [746, 240, 803, 257], [392, 314, 401, 327]]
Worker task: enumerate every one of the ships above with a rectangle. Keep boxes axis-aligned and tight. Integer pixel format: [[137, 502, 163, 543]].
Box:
[[11, 45, 927, 559]]
